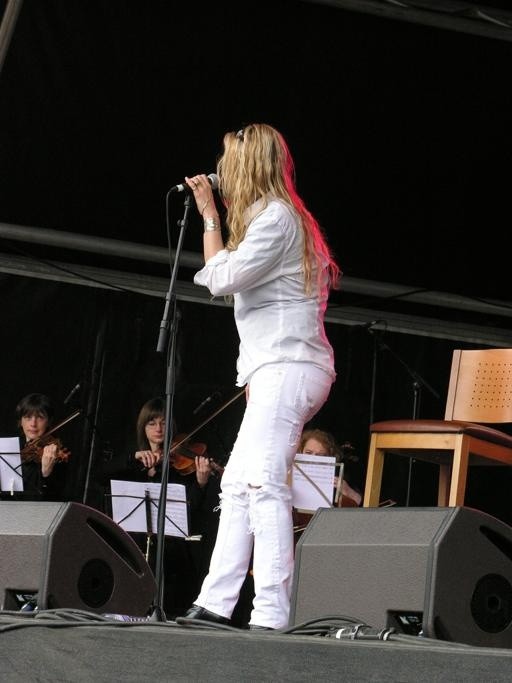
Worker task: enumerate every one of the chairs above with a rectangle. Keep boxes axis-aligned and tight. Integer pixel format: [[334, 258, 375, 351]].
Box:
[[360, 348, 511, 508]]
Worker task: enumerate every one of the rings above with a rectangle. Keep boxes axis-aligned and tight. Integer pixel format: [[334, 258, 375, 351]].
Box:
[[193, 180, 200, 184], [192, 186, 197, 191], [145, 452, 149, 455]]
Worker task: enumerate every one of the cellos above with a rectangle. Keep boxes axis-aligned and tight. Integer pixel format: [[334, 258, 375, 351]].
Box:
[[293, 441, 357, 558]]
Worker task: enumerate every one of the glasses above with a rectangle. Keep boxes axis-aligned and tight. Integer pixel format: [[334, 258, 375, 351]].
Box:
[[235, 129, 244, 142]]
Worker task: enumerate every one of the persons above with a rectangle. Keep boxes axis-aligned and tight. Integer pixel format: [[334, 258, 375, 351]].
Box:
[[94, 396, 212, 619], [182, 122, 344, 632], [300, 423, 362, 507], [0, 392, 71, 502]]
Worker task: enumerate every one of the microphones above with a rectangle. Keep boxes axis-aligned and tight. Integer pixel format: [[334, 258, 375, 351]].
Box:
[[175, 174, 218, 193]]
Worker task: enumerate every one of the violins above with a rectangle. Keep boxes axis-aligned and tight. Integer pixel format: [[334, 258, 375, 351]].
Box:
[[21, 432, 71, 464], [156, 432, 224, 475]]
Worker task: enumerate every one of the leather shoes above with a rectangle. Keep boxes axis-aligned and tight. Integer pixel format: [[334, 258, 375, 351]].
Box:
[[186, 607, 229, 625]]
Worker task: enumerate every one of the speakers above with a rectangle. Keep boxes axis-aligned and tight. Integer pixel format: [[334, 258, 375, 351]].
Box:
[[289, 506, 511, 648], [0, 500, 160, 625]]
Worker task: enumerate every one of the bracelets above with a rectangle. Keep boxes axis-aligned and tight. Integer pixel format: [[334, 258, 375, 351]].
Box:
[[203, 216, 221, 233], [349, 486, 353, 500]]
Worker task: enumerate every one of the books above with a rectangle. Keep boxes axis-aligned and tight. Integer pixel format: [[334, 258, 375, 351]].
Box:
[[292, 453, 335, 513], [110, 478, 189, 539], [0, 436, 24, 493]]
